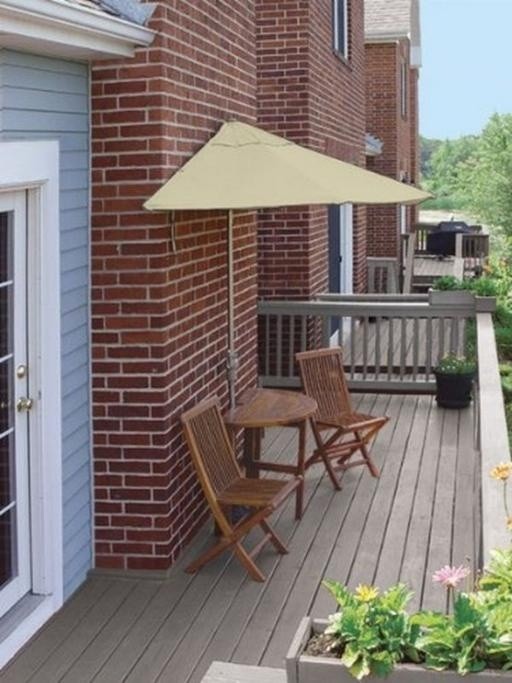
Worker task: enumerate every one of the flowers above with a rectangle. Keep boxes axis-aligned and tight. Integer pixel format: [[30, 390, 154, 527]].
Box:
[[490, 462, 512, 548], [432, 566, 470, 614], [353, 581, 380, 607]]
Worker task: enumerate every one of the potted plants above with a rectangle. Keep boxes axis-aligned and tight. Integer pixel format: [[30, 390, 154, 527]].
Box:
[[427, 274, 475, 305], [473, 277, 497, 313], [433, 355, 476, 407]]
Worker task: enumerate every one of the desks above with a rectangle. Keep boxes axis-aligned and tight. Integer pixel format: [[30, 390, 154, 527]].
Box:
[[214, 388, 317, 535]]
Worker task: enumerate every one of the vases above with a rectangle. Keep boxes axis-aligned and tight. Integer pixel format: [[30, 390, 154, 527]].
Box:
[[287, 616, 511, 683]]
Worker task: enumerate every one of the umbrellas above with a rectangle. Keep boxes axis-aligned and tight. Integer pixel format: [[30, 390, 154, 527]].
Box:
[[142, 118, 436, 410]]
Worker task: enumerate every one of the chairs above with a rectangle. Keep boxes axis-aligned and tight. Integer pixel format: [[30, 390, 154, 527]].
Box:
[[295, 349, 389, 490], [180, 396, 304, 581]]
